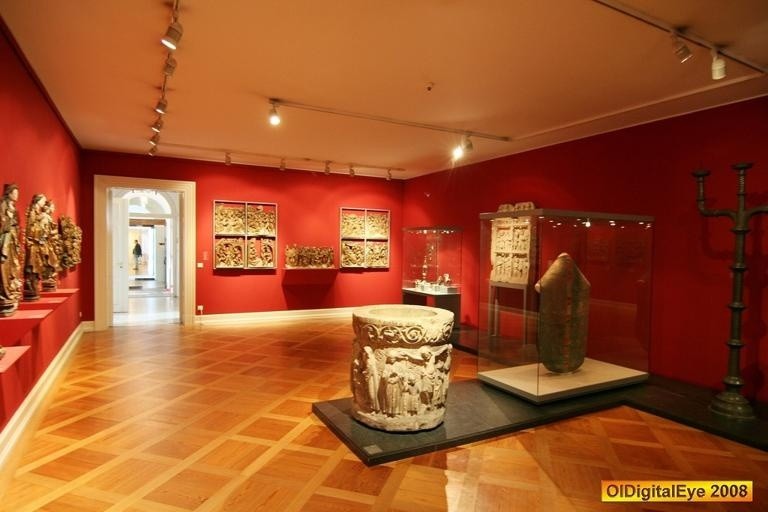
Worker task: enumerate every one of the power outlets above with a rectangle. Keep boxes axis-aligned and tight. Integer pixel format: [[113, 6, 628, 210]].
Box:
[[197, 305, 203, 310]]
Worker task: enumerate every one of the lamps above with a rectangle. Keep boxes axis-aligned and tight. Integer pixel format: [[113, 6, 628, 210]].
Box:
[[267, 104, 284, 126], [669, 32, 728, 82], [220, 152, 395, 181], [148, 17, 184, 157]]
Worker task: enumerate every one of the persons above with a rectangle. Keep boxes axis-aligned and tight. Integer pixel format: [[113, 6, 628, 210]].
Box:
[[57, 213, 83, 269], [40, 200, 64, 280], [285, 243, 336, 268], [24, 193, 48, 296], [132, 240, 143, 270], [0, 181, 25, 308]]
